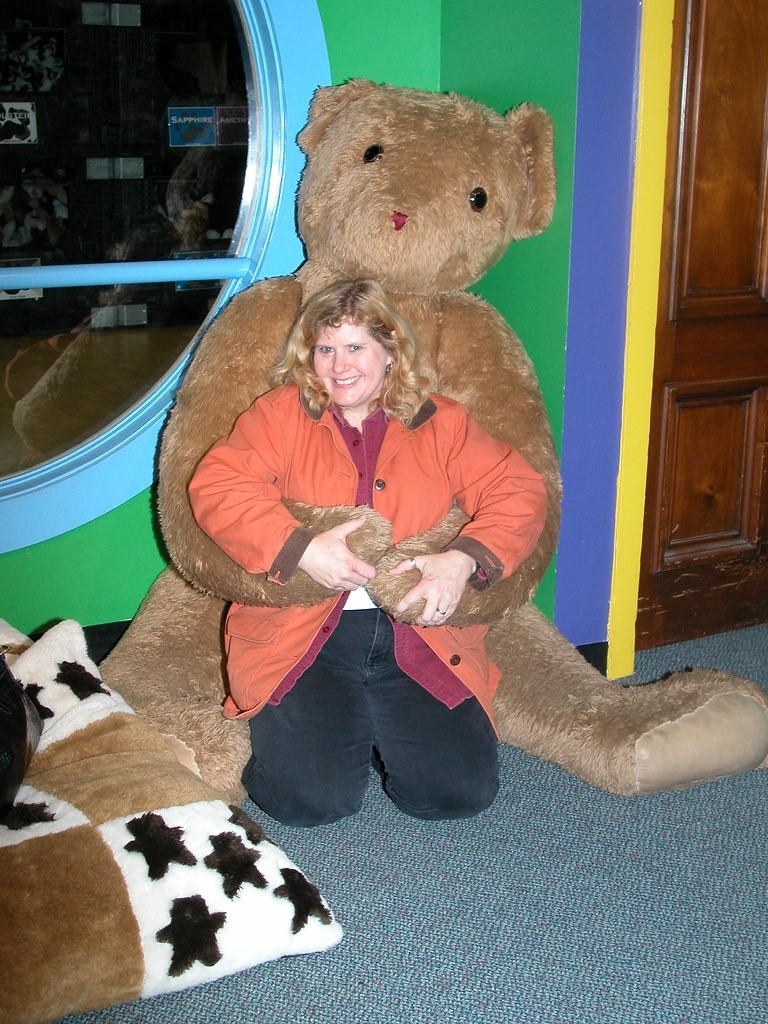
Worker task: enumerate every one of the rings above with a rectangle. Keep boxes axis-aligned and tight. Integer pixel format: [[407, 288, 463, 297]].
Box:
[[437, 608, 446, 615], [410, 557, 416, 569]]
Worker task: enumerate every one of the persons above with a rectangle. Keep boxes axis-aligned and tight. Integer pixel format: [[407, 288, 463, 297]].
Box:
[[188, 278, 548, 829], [70, 219, 170, 333]]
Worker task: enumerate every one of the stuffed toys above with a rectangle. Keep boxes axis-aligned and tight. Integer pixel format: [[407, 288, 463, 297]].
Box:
[[0, 77, 768, 802], [0, 97, 248, 478]]
